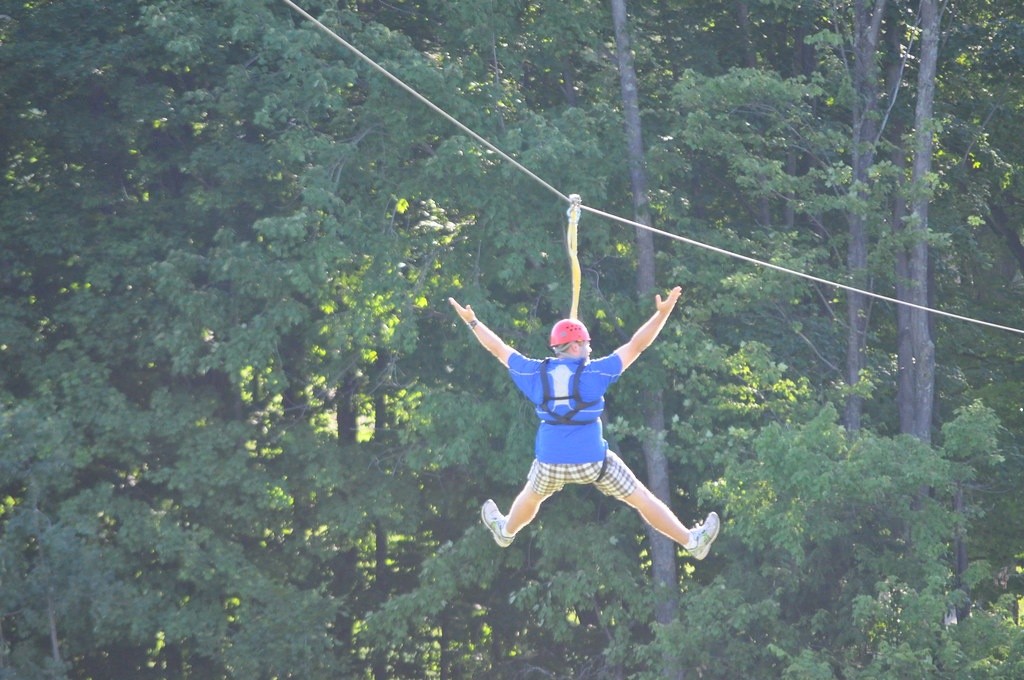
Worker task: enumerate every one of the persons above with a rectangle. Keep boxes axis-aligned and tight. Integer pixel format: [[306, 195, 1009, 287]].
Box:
[[449, 286, 721, 562]]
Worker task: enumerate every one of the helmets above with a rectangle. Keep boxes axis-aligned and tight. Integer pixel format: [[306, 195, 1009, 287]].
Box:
[[549, 318, 591, 347]]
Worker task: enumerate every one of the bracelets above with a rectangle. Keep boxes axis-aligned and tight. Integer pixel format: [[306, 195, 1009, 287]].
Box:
[[468, 319, 479, 329]]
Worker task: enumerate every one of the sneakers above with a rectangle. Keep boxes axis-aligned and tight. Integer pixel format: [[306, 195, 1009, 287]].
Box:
[[481, 498, 516, 548], [686, 511, 720, 561]]
